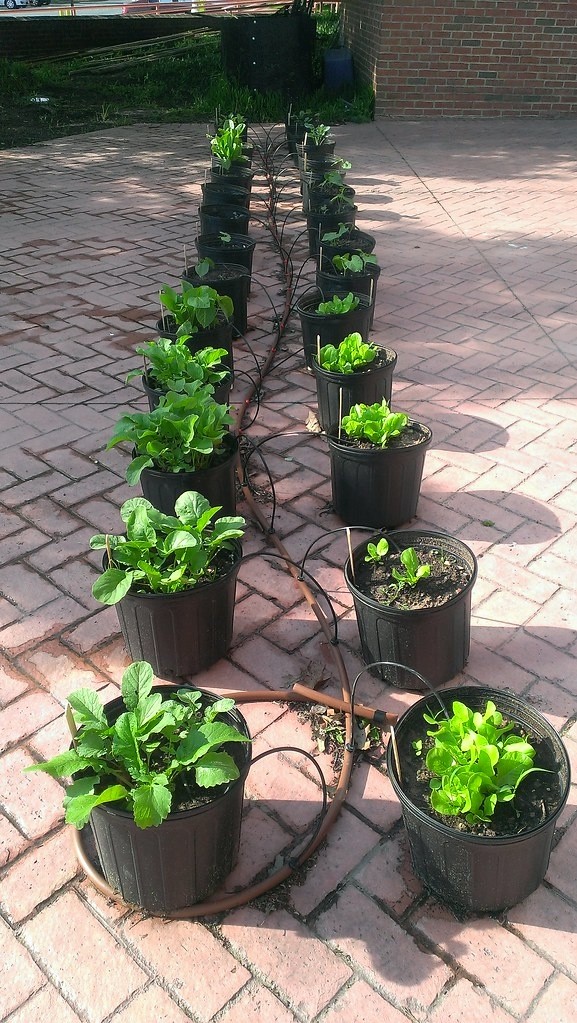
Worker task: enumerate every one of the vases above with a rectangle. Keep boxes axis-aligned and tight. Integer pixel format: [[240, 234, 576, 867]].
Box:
[[196, 204, 251, 236], [201, 182, 248, 205]]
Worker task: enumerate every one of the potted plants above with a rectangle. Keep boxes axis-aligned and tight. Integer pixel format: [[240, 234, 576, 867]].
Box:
[[386, 685, 571, 912], [125, 102, 257, 519], [88, 490, 243, 681], [21, 660, 253, 912], [343, 530, 478, 691], [285, 102, 433, 530]]
